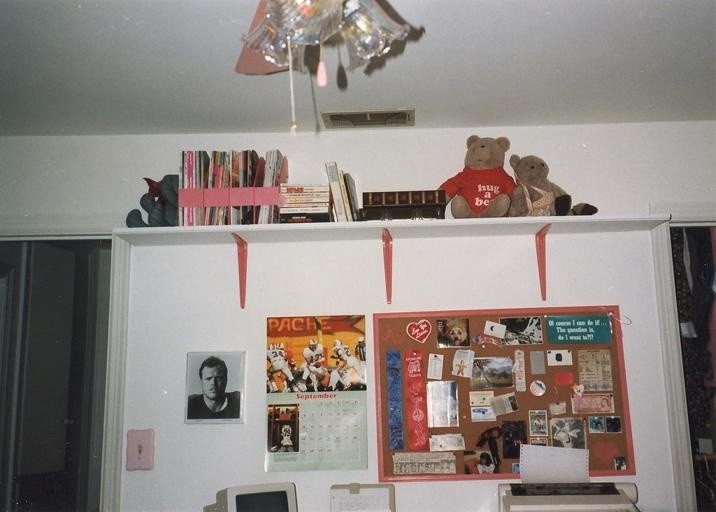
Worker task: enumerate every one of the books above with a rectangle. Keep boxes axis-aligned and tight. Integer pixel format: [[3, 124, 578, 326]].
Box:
[[329, 163, 358, 221], [279, 182, 331, 222], [361, 189, 446, 219], [180, 147, 288, 225]]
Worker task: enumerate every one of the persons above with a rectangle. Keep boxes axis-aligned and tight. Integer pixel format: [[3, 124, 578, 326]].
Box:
[[615, 458, 627, 473], [534, 415, 545, 434], [591, 420, 602, 433], [477, 452, 495, 475], [185, 355, 240, 421], [265, 315, 367, 393]]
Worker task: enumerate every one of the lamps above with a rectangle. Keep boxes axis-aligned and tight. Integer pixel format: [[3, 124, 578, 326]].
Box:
[[235, 0, 422, 133]]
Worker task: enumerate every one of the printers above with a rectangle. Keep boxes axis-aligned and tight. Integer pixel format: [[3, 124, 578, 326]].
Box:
[[497, 482, 640, 512]]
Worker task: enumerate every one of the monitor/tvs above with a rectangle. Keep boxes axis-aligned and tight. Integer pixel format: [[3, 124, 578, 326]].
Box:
[[202, 480, 298, 511]]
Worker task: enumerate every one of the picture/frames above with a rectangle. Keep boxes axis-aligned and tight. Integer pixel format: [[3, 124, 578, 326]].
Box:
[[182, 349, 245, 425], [373, 305, 638, 484]]
